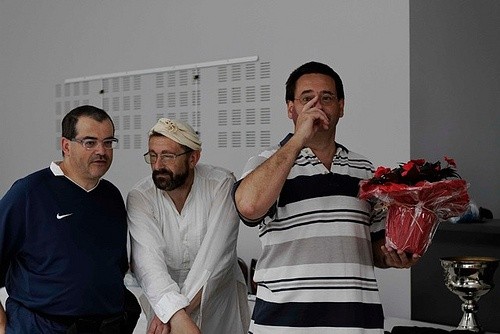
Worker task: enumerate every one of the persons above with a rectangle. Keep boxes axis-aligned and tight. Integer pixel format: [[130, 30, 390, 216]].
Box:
[[0, 105, 125, 334], [231, 61, 422, 334], [126, 118, 250, 334]]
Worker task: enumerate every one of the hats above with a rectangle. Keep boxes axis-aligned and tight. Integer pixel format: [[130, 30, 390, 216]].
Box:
[[151, 118, 202, 150]]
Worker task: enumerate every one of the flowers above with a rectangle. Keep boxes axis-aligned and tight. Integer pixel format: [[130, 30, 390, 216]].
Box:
[[360, 156, 473, 256]]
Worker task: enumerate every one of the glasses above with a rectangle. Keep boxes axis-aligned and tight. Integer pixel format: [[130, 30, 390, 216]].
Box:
[[144, 151, 193, 164], [71, 137, 118, 150], [294, 94, 338, 105]]
